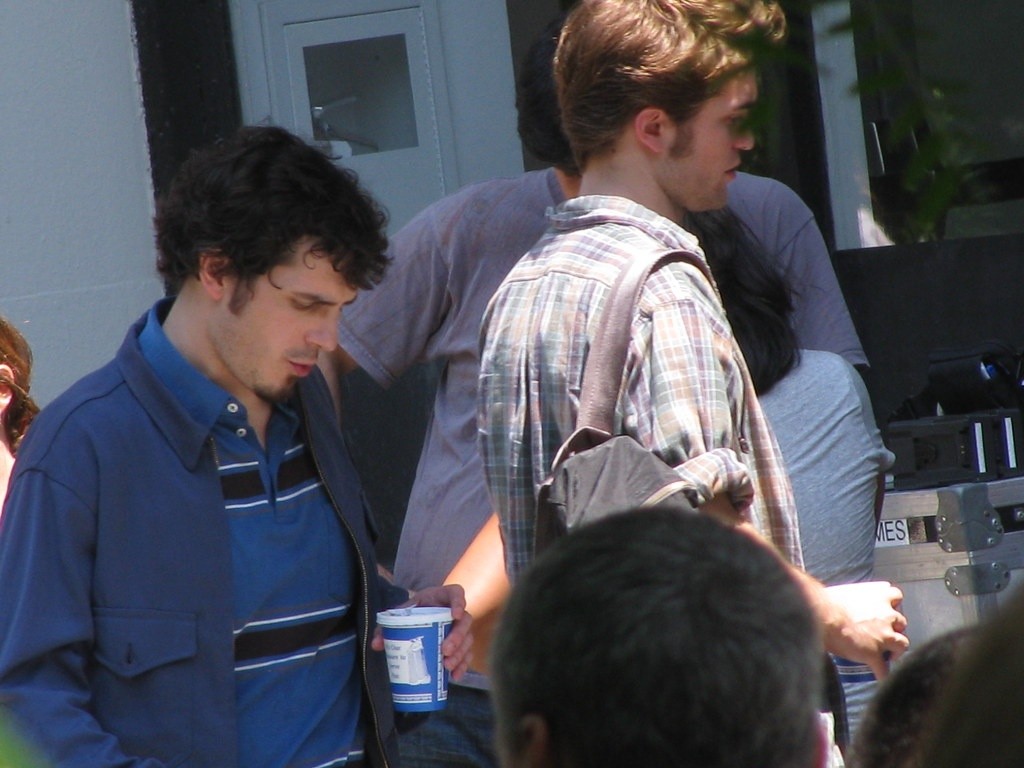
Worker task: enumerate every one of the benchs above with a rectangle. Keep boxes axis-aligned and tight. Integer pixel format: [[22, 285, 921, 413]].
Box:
[[342, 232, 1024, 574]]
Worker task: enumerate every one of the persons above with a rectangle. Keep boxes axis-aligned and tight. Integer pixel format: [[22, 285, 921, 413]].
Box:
[[1, 123, 475, 768], [846, 589, 1024, 768], [441, 249, 897, 747], [476, 0, 909, 750], [318, 23, 875, 768], [490, 503, 831, 768], [0, 318, 45, 768]]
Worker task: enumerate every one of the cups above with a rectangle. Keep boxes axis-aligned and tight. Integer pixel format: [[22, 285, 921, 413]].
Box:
[[375, 605, 454, 713], [832, 648, 890, 746]]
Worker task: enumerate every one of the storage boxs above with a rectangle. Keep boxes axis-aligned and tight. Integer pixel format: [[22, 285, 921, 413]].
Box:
[[871, 476, 1024, 665]]
